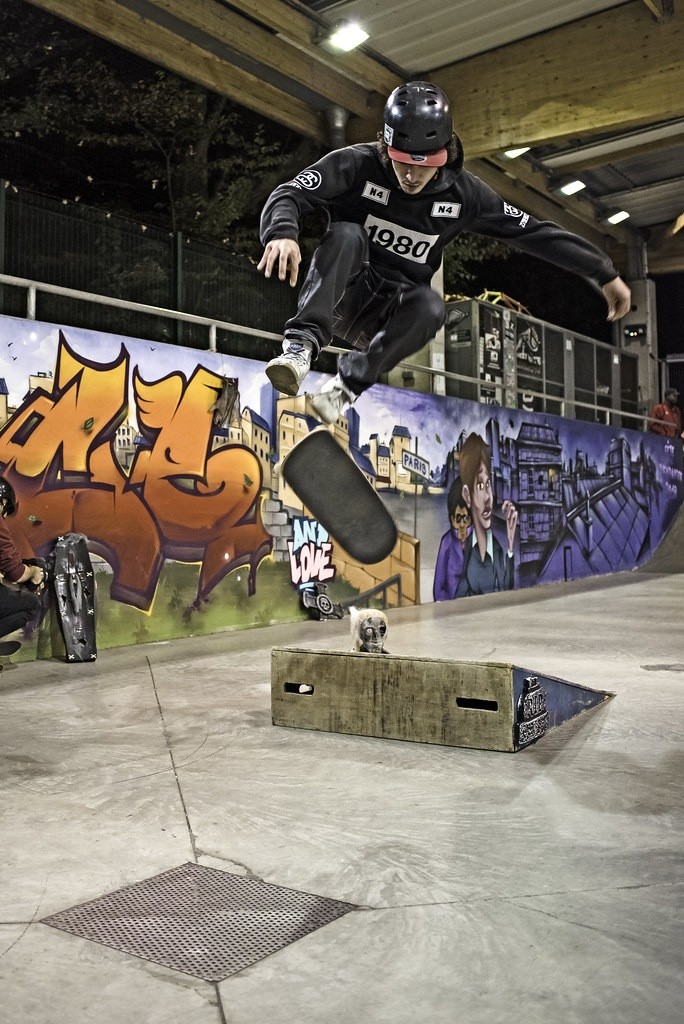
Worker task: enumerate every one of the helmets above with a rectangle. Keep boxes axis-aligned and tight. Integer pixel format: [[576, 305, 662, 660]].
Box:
[[0, 477, 16, 514], [383, 81, 453, 153]]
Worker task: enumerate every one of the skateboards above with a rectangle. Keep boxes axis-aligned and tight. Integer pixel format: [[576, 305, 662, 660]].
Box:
[[279, 427, 398, 564], [52, 532, 97, 664]]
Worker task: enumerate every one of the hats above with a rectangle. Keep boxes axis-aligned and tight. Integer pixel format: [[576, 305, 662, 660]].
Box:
[[665, 388, 680, 396], [388, 145, 448, 167]]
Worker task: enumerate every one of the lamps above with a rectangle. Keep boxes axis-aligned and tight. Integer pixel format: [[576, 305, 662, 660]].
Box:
[[312, 18, 370, 56], [497, 144, 531, 162], [596, 206, 630, 226], [553, 172, 586, 199]]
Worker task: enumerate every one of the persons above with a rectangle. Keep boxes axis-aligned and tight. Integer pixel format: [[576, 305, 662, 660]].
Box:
[[0, 474, 44, 671], [256, 83, 630, 425], [650, 389, 682, 437]]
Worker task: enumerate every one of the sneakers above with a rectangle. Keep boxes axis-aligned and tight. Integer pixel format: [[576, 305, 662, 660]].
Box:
[[264, 339, 311, 396], [310, 372, 359, 424]]
